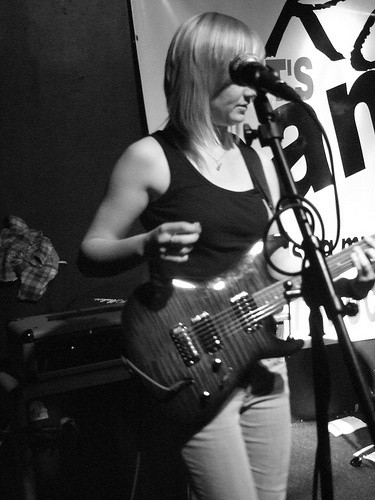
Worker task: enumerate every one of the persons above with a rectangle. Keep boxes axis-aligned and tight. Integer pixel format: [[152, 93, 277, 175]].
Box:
[[74, 11, 375, 500]]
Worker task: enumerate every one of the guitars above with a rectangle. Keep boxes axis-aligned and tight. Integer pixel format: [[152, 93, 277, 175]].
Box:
[[124, 234, 374, 423]]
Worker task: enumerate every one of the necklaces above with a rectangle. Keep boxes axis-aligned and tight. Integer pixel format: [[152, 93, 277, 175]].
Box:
[[191, 138, 233, 170]]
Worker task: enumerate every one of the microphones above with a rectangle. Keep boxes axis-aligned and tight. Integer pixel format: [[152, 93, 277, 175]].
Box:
[[229, 52, 301, 104]]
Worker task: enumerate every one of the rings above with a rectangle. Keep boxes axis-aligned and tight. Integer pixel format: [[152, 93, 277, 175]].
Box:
[[168, 233, 175, 246]]
